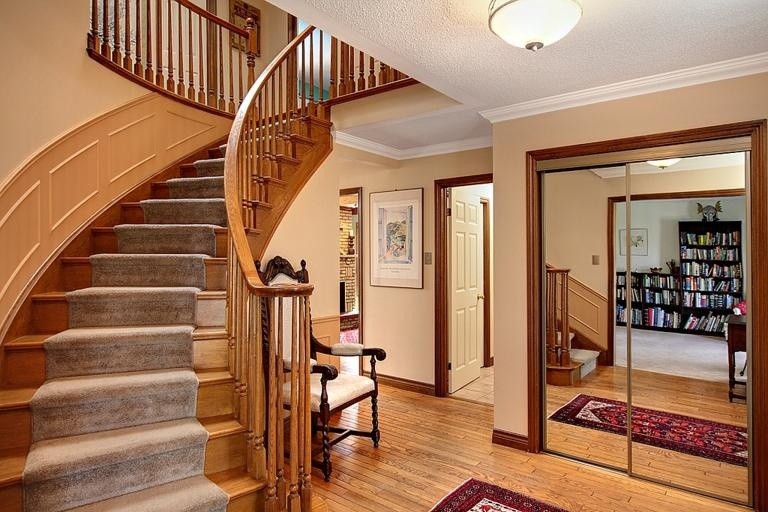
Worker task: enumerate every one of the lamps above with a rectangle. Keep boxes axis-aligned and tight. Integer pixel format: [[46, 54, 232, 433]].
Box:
[[487, 0, 583, 52]]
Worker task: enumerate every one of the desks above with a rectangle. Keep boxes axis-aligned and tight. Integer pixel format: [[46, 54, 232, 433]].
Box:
[[725, 314, 748, 401]]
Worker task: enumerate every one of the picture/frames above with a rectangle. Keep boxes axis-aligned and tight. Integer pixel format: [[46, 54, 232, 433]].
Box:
[[368, 188, 424, 289], [228, 0, 261, 56], [619, 229, 649, 256]]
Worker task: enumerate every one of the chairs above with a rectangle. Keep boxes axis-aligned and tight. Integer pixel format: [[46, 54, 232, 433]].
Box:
[[256, 257, 386, 483]]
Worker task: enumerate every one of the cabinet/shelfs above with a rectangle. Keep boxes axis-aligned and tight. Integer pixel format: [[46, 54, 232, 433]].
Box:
[[617, 222, 743, 336]]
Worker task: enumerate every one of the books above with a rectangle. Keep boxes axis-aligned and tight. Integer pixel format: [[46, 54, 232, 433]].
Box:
[[616, 275, 680, 328], [682, 232, 743, 333]]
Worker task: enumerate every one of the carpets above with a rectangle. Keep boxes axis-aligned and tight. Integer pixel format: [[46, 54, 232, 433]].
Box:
[[430, 479, 566, 512], [550, 391, 750, 470]]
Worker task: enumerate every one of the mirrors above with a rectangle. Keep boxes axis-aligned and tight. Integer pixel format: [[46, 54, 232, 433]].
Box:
[[539, 150, 753, 508]]
